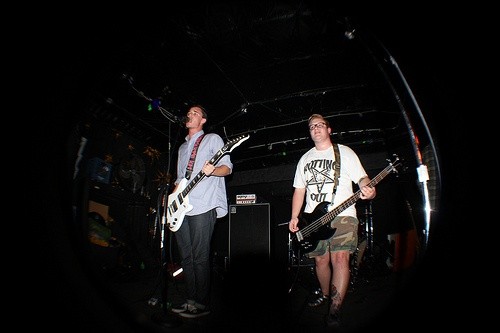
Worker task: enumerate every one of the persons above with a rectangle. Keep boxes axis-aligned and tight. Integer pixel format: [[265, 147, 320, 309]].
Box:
[[289, 113, 376, 328], [160, 104, 232, 318]]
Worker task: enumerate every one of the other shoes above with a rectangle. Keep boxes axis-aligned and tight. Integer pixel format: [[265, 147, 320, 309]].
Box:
[[327, 310, 344, 328], [305, 295, 330, 312]]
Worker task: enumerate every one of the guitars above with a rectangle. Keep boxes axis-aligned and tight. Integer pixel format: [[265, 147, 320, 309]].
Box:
[[292, 153, 402, 254], [166, 135, 251, 232]]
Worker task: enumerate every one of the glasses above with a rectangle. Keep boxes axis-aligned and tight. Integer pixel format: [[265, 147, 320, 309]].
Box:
[[308, 122, 328, 130]]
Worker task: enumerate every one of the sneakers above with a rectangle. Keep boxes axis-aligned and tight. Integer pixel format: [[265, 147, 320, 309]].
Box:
[[171, 303, 210, 318]]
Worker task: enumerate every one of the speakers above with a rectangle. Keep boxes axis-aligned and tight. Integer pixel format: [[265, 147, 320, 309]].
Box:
[[227, 202, 271, 272]]
